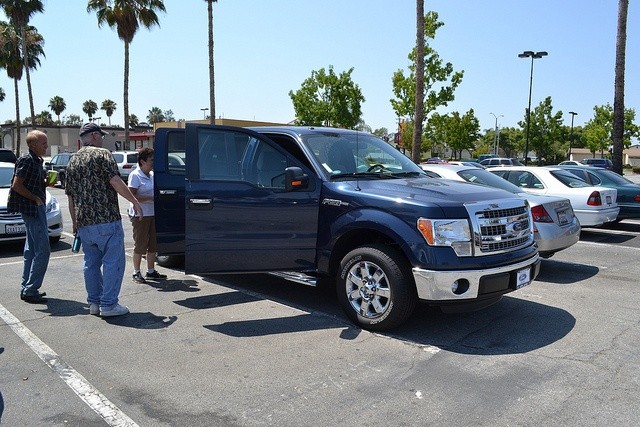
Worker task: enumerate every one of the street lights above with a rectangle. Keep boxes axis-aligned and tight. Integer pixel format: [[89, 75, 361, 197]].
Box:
[[489, 113, 504, 154], [94, 117, 102, 125], [569, 112, 578, 160], [200, 107, 209, 120], [518, 51, 548, 163]]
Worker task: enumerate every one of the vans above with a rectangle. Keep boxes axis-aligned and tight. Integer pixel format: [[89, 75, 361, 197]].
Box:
[[580, 157, 613, 171], [527, 156, 539, 162], [476, 154, 497, 164], [479, 157, 520, 166]]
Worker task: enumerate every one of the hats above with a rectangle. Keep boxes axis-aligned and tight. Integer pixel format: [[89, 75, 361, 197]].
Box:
[[79, 122, 109, 137]]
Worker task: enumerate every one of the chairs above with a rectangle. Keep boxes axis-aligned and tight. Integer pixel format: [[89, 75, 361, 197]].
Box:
[[523, 176, 538, 188], [328, 138, 356, 173], [510, 173, 520, 187], [257, 151, 281, 185]]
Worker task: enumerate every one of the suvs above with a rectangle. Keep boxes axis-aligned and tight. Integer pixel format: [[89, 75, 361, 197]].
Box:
[[0, 149, 17, 162], [144, 124, 542, 332], [43, 153, 76, 188], [112, 151, 140, 182]]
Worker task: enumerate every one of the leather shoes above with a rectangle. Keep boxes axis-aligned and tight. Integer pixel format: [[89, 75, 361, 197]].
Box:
[[20, 292, 48, 303]]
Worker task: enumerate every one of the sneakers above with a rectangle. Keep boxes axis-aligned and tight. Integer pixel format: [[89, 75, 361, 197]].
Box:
[[145, 270, 167, 280], [100, 304, 129, 317], [90, 304, 100, 315], [132, 272, 146, 284]]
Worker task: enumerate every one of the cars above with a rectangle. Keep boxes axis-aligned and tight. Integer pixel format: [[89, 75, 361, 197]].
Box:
[[448, 162, 476, 167], [0, 161, 63, 242], [560, 167, 639, 219], [416, 163, 581, 259], [471, 162, 486, 169], [480, 166, 622, 229], [419, 161, 449, 165]]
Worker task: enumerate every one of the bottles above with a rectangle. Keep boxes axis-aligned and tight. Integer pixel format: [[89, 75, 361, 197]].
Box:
[[72, 229, 81, 253]]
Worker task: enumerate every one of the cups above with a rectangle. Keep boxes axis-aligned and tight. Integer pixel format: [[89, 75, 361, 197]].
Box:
[[48, 170, 57, 183]]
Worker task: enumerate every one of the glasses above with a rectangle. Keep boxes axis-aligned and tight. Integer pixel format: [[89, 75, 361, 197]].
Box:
[[91, 132, 104, 140]]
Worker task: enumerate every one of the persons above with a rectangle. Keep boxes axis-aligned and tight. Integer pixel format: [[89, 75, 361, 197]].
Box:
[[128, 147, 167, 284], [65, 123, 143, 317], [7, 130, 58, 304]]
